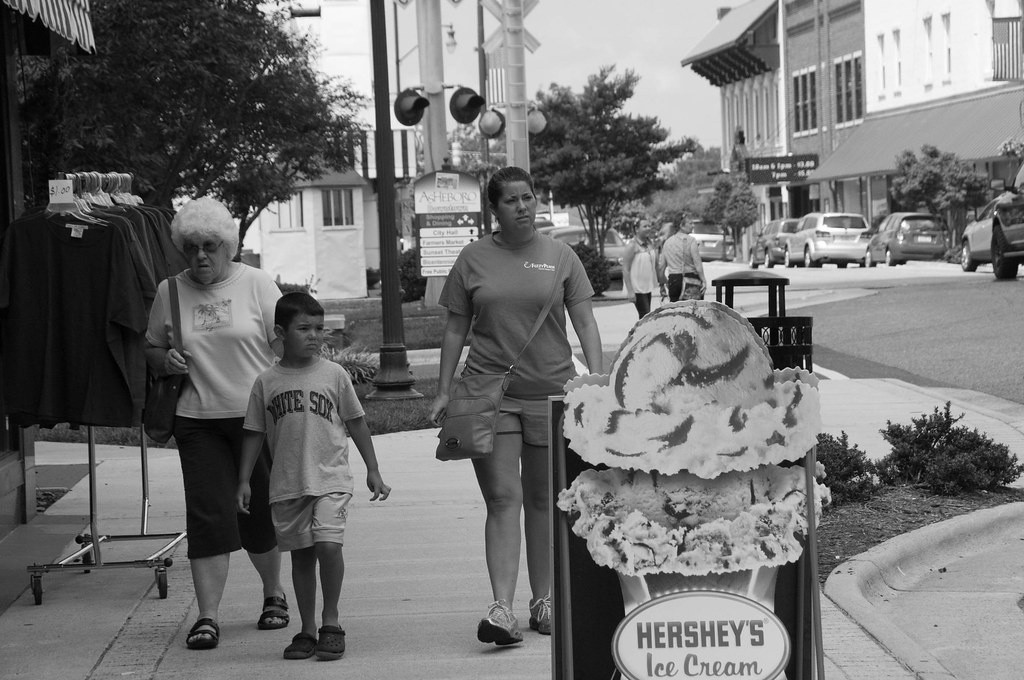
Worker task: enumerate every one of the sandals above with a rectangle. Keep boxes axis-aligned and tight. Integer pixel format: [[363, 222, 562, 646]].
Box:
[[280, 633, 318, 660], [256, 591, 291, 630], [314, 622, 346, 661], [186, 616, 220, 650]]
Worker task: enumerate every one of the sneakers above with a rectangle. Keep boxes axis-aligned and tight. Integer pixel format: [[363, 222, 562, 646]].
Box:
[[478, 606, 524, 646], [528, 598, 553, 635]]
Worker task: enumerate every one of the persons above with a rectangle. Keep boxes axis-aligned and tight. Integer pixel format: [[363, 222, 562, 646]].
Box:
[[141, 194, 288, 651], [234, 292, 392, 662], [423, 164, 603, 648], [621, 216, 667, 322], [655, 211, 708, 302]]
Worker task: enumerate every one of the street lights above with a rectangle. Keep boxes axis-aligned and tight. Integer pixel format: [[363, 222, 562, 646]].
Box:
[[475, 98, 506, 237]]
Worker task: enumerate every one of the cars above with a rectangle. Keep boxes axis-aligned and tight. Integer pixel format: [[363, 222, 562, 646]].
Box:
[[864, 211, 949, 267], [784, 211, 870, 268], [960, 191, 1006, 272], [749, 218, 800, 269], [654, 222, 674, 254], [548, 226, 629, 282], [989, 163, 1024, 280], [687, 218, 735, 261]]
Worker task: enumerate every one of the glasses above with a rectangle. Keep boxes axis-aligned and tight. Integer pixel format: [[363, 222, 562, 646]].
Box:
[[184, 239, 224, 255]]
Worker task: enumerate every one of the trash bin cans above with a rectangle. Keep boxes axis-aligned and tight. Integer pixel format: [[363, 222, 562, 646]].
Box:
[[711, 271, 813, 373]]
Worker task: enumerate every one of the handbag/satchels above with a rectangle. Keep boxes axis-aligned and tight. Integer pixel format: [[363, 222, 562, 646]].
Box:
[[140, 357, 184, 445], [679, 277, 703, 300], [435, 369, 510, 461]]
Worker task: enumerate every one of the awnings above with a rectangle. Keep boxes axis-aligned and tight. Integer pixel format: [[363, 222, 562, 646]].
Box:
[[1, 0, 97, 61]]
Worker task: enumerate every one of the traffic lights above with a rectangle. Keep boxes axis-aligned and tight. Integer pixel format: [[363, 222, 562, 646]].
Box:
[[394, 89, 431, 127], [449, 87, 483, 125]]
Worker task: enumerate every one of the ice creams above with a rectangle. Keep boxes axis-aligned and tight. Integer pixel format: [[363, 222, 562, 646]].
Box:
[[555, 300, 832, 618]]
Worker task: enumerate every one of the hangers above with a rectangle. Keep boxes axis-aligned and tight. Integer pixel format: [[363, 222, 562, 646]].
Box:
[[32, 170, 145, 228]]
